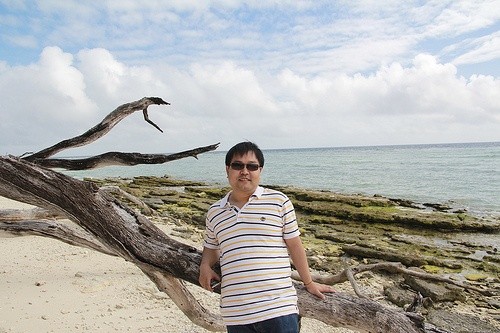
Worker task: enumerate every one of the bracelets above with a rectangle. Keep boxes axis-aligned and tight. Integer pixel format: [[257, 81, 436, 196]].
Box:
[[305, 280, 313, 285]]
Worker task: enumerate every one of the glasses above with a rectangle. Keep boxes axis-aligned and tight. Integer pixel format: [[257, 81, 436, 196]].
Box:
[[228, 162, 261, 171]]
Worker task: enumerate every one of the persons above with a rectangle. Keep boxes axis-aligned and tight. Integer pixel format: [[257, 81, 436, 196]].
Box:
[[199, 142, 336, 333]]
